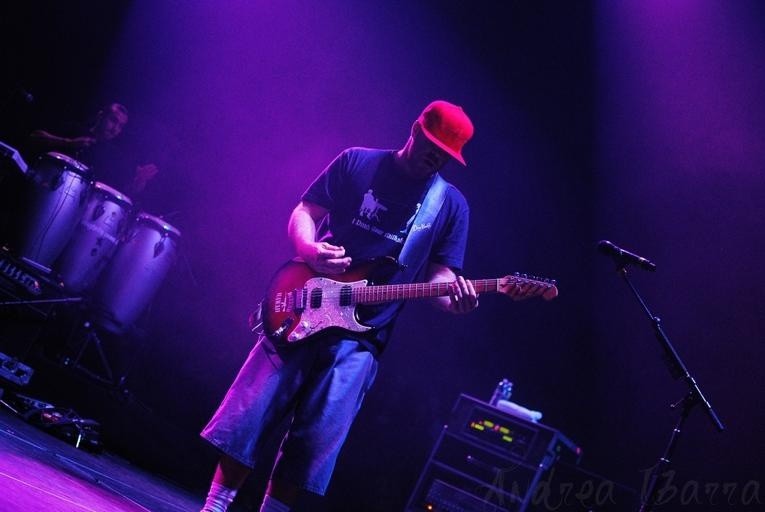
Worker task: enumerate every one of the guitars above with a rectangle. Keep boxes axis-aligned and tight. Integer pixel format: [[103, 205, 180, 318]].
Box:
[[262, 257, 558, 357]]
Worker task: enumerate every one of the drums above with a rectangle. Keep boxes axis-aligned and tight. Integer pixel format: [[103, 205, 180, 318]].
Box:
[[49, 175, 134, 311], [9, 150, 91, 282], [85, 210, 183, 336]]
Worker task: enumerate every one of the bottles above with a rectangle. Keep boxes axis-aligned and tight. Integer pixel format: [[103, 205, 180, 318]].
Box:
[[489, 379, 513, 406]]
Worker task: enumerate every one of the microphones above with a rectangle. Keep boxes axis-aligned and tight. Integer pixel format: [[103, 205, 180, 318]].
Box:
[[596, 240, 657, 271]]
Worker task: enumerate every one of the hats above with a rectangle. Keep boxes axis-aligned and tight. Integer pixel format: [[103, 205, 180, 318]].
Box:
[[419, 100, 476, 168]]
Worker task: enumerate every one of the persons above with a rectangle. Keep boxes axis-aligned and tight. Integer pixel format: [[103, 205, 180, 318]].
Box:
[[200, 99, 482, 512], [25, 98, 157, 200]]
